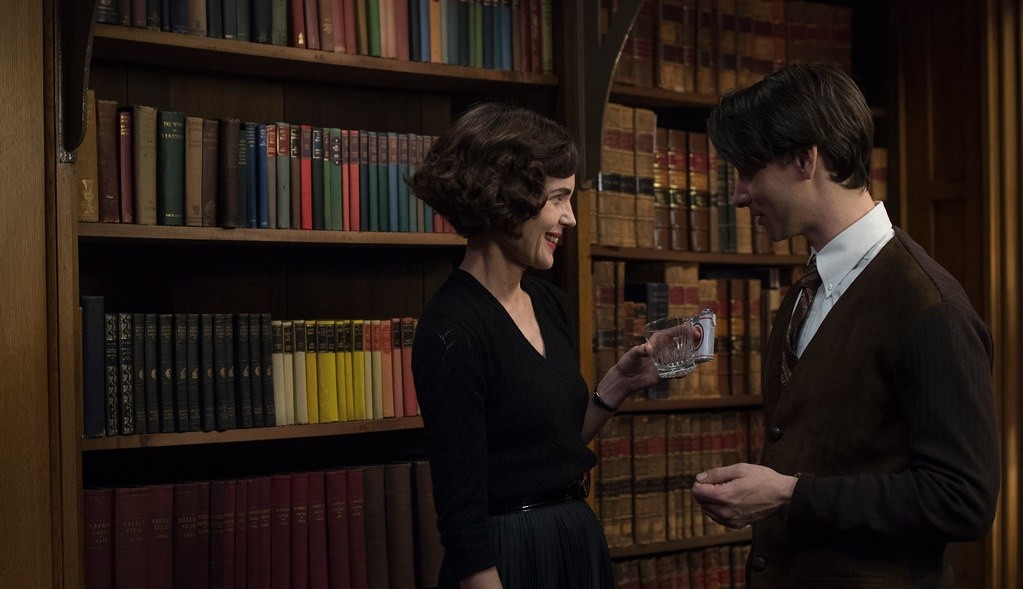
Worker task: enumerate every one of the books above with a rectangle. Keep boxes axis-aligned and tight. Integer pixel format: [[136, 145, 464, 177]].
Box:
[[78, 0, 885, 589]]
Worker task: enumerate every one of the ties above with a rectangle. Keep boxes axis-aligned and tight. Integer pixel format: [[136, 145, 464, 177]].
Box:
[[767, 263, 820, 397]]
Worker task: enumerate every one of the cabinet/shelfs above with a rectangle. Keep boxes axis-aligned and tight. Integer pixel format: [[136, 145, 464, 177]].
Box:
[[40, 0, 907, 589]]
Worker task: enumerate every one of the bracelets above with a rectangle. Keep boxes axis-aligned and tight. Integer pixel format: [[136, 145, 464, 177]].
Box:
[[590, 380, 621, 415]]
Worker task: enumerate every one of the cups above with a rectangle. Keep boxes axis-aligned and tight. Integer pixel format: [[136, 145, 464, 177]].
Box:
[[642, 316, 696, 378]]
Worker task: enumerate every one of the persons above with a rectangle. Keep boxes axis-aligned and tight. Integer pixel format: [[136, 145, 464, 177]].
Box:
[[403, 105, 704, 589], [693, 61, 1002, 589]]
